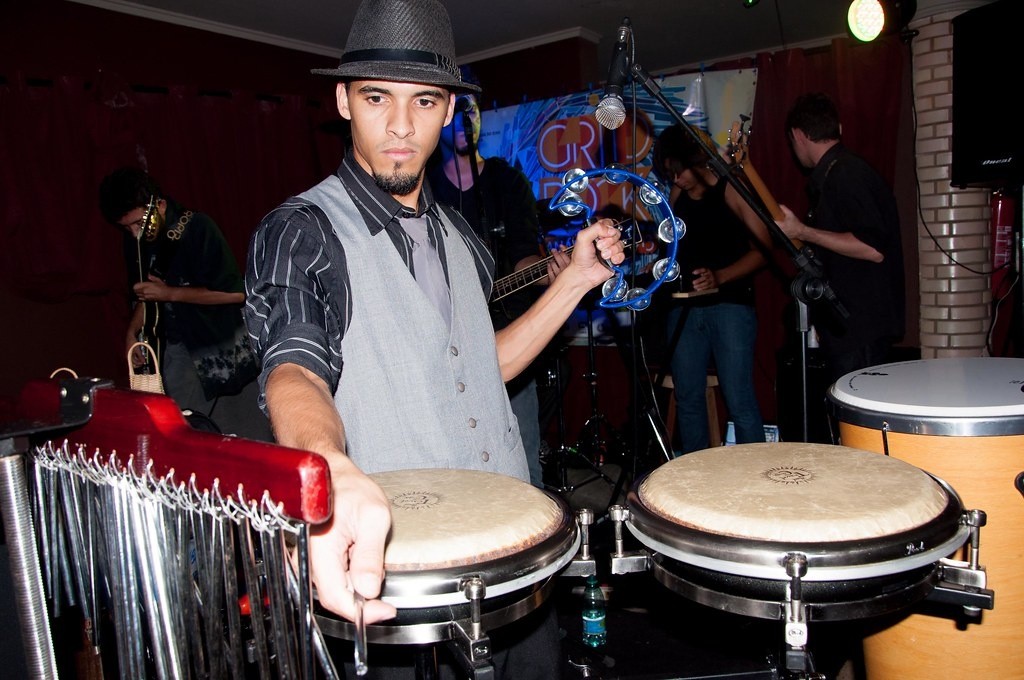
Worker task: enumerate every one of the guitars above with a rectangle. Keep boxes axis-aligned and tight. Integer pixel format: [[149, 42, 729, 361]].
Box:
[[727, 113, 805, 252], [132, 193, 163, 374], [489, 216, 643, 303]]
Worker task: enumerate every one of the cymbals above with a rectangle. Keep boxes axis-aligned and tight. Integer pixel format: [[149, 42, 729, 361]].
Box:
[[547, 162, 686, 312]]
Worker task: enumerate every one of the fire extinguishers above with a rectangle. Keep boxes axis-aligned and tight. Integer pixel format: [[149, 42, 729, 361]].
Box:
[[989, 186, 1016, 297]]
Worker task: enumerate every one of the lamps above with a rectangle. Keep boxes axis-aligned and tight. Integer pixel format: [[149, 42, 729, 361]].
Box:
[[847, 0, 920, 44]]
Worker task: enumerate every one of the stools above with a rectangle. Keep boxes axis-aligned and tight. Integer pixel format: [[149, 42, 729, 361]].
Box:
[[653, 373, 721, 448]]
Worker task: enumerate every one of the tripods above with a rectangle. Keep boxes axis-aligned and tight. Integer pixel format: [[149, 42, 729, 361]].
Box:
[[539, 307, 635, 500]]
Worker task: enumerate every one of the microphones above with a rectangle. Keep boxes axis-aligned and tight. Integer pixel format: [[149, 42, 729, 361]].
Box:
[[452, 95, 473, 116], [596, 17, 631, 130]]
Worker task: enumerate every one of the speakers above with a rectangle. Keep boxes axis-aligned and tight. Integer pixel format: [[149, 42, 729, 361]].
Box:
[[949, 0, 1024, 190]]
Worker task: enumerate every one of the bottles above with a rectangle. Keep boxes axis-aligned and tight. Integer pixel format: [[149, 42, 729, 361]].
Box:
[[582, 574, 608, 648], [724, 422, 736, 446]]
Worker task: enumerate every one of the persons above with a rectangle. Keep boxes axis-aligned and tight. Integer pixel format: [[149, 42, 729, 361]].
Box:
[[591, 123, 773, 453], [98, 165, 276, 441], [426, 66, 573, 491], [242, 0, 625, 680], [772, 93, 905, 446]]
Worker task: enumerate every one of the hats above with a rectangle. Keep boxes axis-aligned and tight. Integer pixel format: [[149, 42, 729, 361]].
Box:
[[309, 1, 486, 97]]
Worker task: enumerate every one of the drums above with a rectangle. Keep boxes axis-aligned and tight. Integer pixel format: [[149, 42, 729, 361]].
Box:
[[627, 441, 967, 644], [291, 466, 580, 667], [824, 355, 1024, 680]]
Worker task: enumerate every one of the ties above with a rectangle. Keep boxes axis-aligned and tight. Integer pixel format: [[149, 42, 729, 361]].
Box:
[[400, 215, 452, 326]]
